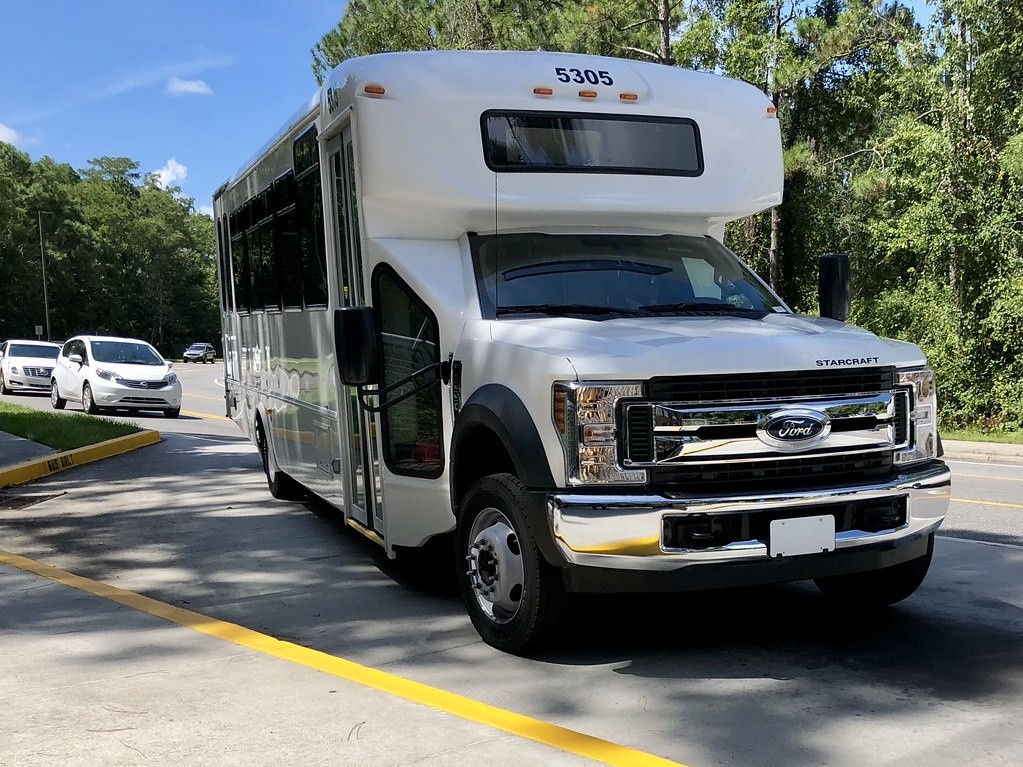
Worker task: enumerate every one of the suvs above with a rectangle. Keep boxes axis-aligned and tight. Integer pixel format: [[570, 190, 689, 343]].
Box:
[[183, 342, 217, 364]]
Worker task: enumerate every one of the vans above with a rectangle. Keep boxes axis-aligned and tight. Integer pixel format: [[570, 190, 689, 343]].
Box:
[[211, 47, 955, 655]]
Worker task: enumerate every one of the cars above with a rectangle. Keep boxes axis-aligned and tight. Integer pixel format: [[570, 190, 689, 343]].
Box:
[[49, 335, 182, 419], [0, 339, 63, 396]]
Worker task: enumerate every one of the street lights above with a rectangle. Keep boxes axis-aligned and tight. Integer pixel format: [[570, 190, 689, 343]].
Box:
[[38, 209, 55, 341]]
